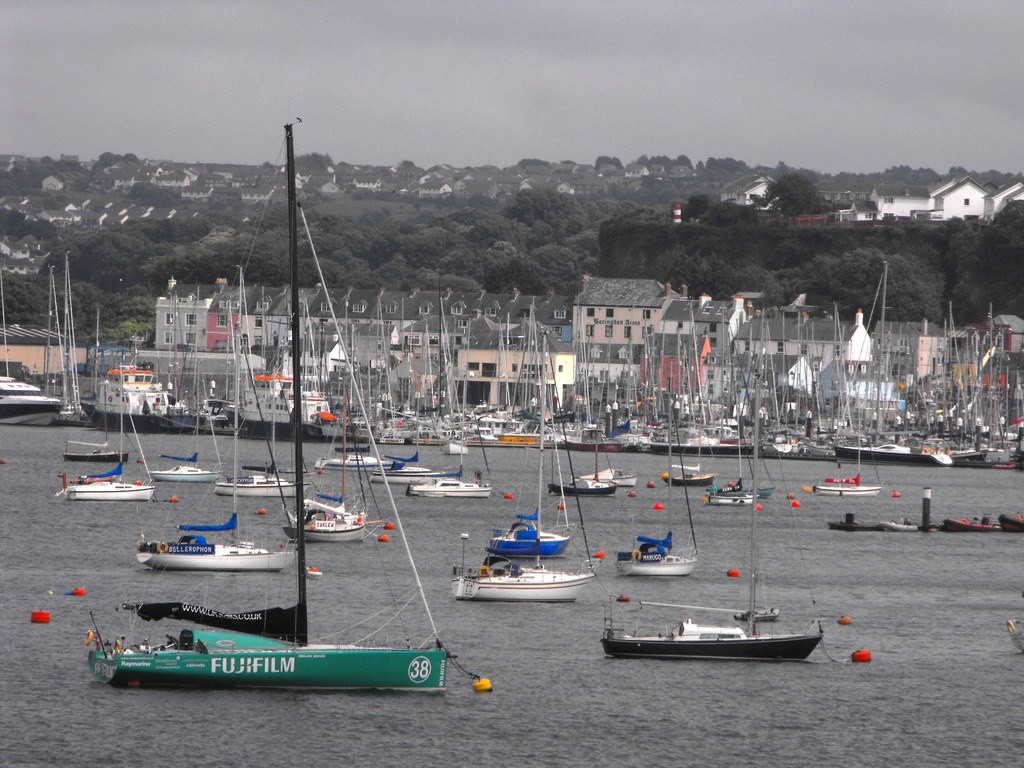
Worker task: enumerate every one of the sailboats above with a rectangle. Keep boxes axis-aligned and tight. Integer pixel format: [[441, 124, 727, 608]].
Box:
[[127, 259, 299, 572], [615, 399, 704, 577], [450, 326, 602, 605], [79, 117, 452, 695], [595, 310, 830, 661], [0, 242, 1023, 559]]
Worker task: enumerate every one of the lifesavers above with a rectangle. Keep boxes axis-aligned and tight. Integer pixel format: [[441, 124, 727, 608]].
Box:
[[478, 565, 490, 576], [84, 629, 95, 646], [1006, 620, 1016, 633], [156, 541, 169, 553], [632, 550, 642, 561]]
